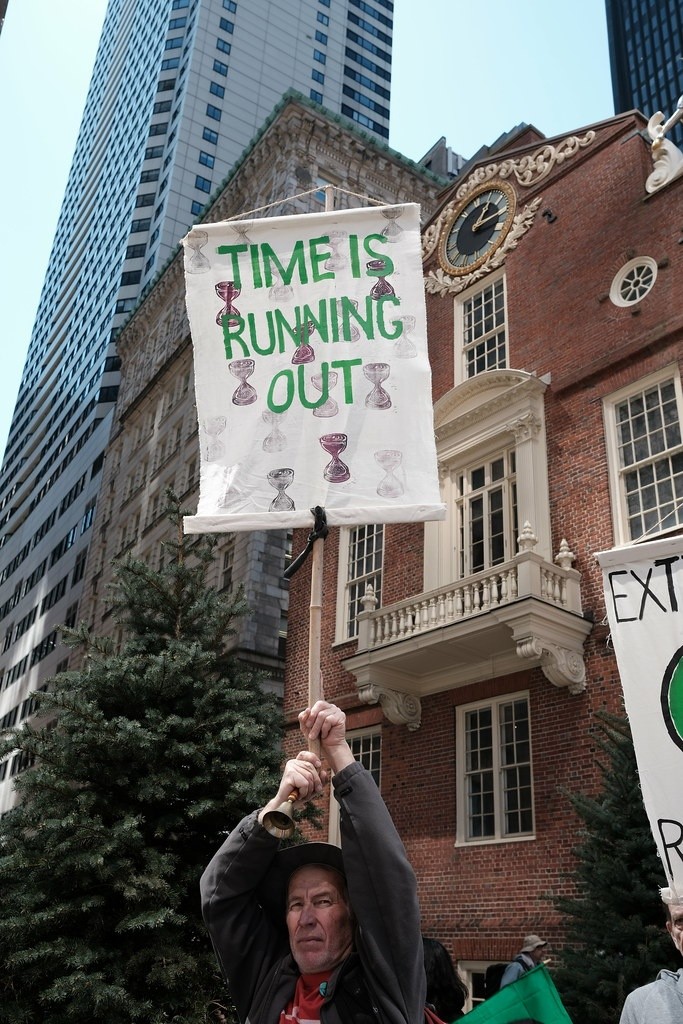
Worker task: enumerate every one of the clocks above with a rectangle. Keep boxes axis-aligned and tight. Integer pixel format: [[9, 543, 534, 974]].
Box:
[[446, 188, 509, 268]]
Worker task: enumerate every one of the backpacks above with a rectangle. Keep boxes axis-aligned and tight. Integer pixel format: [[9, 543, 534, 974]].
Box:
[[485, 958, 540, 1000]]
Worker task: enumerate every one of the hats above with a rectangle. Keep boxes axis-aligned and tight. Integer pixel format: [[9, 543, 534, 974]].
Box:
[[257, 842, 348, 914], [521, 935, 548, 952]]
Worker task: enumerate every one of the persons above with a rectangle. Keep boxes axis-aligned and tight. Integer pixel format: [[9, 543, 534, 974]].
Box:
[[501, 935, 547, 988], [423, 937, 469, 1024], [200, 669, 426, 1024], [620, 904, 683, 1024]]
